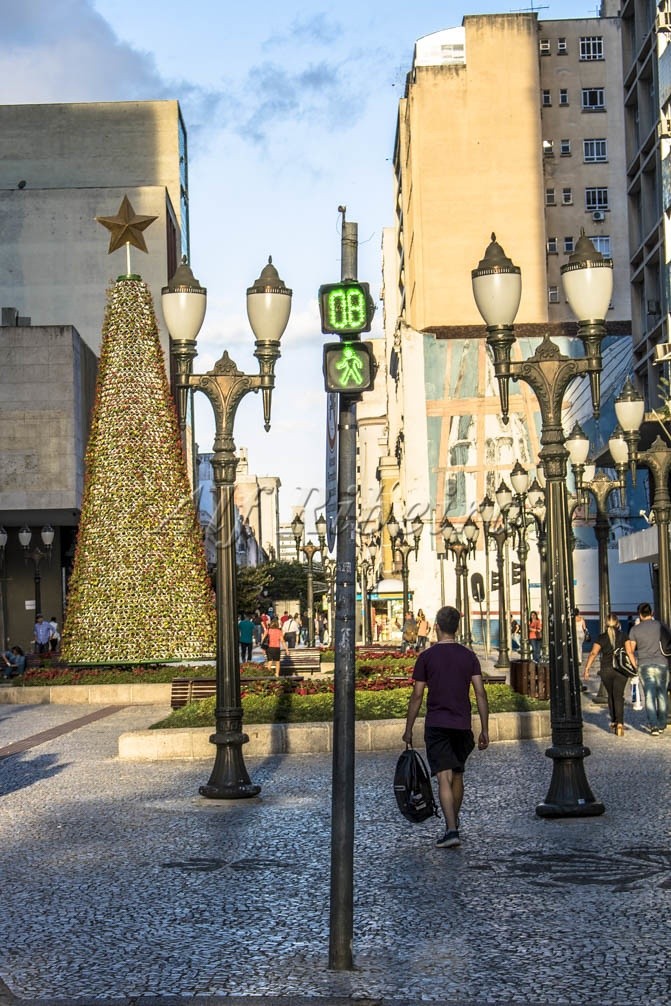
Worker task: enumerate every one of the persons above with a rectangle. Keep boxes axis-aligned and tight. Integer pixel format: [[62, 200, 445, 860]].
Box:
[[402, 606, 491, 847], [527, 611, 542, 664], [34, 614, 60, 655], [574, 607, 587, 666], [394, 609, 438, 651], [261, 618, 289, 679], [510, 614, 521, 651], [583, 614, 630, 737], [238, 612, 256, 664], [628, 603, 671, 735], [237, 609, 329, 648], [2, 646, 26, 680]]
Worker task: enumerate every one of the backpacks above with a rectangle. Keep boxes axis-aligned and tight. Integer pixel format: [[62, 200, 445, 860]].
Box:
[[393, 742, 437, 823]]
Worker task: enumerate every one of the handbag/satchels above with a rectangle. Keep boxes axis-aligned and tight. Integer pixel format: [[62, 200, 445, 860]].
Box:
[[261, 627, 270, 650], [284, 632, 287, 641], [584, 630, 590, 641], [613, 646, 638, 677], [403, 619, 417, 643]]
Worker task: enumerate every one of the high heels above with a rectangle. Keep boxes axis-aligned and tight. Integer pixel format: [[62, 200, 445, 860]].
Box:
[[610, 725, 624, 736]]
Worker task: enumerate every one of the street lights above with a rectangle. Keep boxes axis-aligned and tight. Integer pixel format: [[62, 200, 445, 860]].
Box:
[[318, 544, 337, 651], [291, 512, 326, 647], [355, 541, 377, 646], [386, 513, 426, 627], [156, 255, 294, 800], [472, 224, 616, 818], [439, 460, 546, 668], [611, 364, 670, 645], [560, 417, 636, 637]]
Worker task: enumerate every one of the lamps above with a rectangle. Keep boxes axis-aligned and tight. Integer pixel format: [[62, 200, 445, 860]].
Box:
[[592, 210, 604, 221], [656, 118, 671, 139], [654, 344, 671, 364], [656, 11, 671, 32]]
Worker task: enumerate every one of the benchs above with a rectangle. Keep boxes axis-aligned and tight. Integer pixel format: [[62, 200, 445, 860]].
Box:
[[264, 650, 322, 676], [359, 647, 398, 654], [171, 677, 305, 713], [388, 675, 508, 683], [1, 654, 58, 676]]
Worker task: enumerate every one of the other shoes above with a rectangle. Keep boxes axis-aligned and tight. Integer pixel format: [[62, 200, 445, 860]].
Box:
[[652, 729, 665, 735], [435, 818, 462, 848]]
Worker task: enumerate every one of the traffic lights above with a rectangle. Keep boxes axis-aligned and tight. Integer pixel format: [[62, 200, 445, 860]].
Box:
[[316, 279, 374, 335], [322, 339, 377, 393]]
